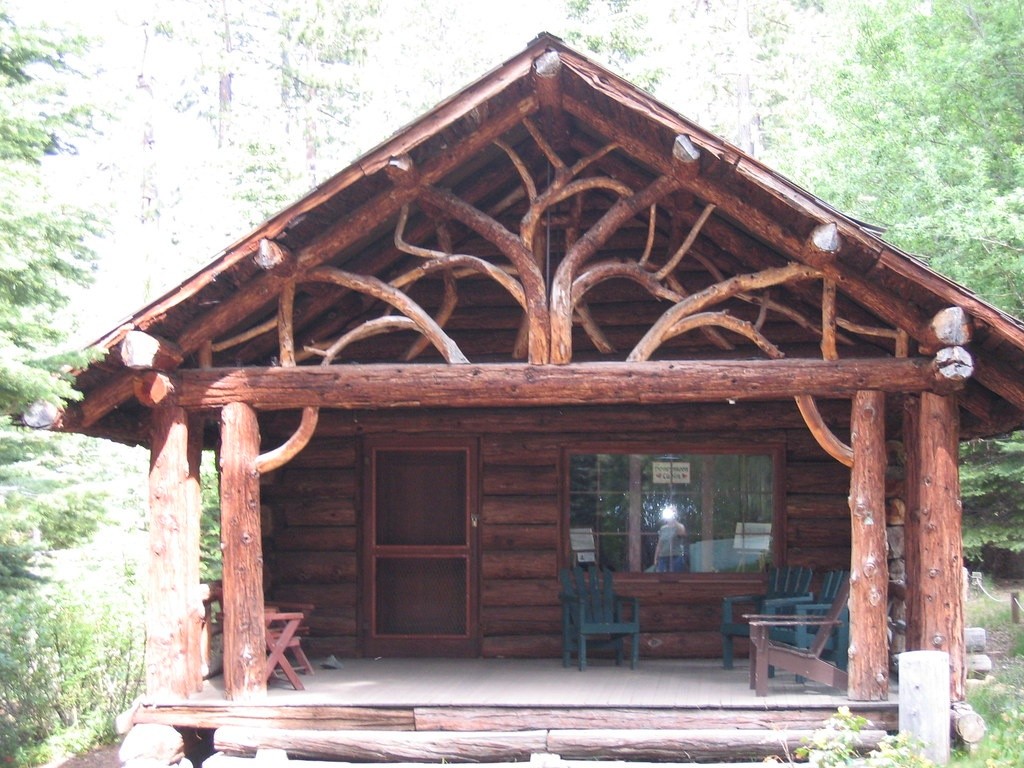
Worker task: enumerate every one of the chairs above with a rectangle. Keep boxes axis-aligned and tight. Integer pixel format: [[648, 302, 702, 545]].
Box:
[[216, 584, 318, 691], [720, 562, 851, 684], [559, 565, 641, 669]]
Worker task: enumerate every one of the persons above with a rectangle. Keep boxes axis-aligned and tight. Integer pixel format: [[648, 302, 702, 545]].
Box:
[[653, 506, 686, 572]]
